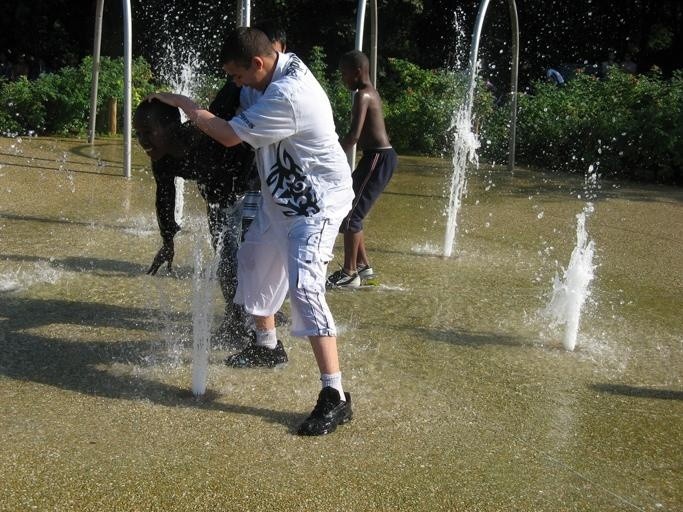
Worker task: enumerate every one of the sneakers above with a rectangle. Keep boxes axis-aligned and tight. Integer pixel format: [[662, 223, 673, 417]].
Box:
[[355, 261, 374, 278], [323, 267, 362, 290], [223, 337, 288, 370], [296, 384, 355, 436]]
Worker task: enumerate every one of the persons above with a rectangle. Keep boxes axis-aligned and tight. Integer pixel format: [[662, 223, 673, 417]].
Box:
[[131, 96, 265, 348], [321, 50, 400, 289], [207, 17, 297, 243], [139, 25, 358, 439]]
